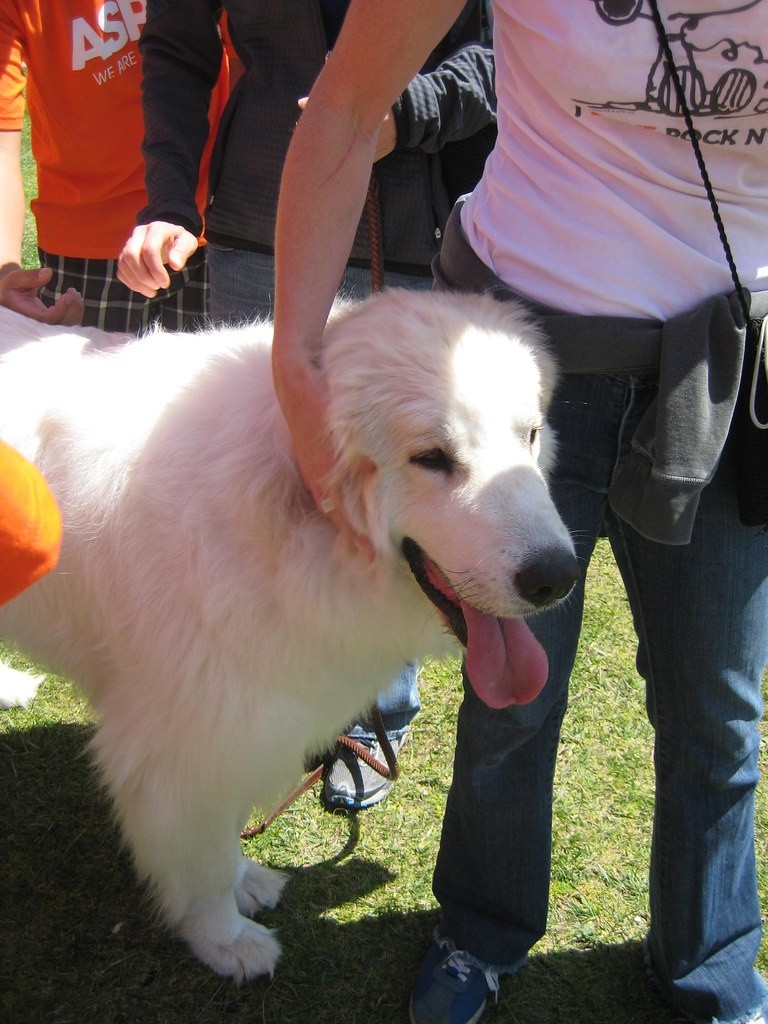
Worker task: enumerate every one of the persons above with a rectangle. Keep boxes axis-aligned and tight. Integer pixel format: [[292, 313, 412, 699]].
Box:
[[270, 0, 768, 1024], [0, 0, 499, 812]]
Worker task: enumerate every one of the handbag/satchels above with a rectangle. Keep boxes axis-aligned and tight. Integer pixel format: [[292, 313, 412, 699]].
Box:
[[724, 315, 768, 527]]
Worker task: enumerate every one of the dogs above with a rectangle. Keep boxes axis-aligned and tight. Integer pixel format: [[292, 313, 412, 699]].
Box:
[[1, 286, 589, 984]]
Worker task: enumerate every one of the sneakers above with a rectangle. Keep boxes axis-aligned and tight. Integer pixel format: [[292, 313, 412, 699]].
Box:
[[409, 938, 499, 1024], [324, 724, 408, 810]]
